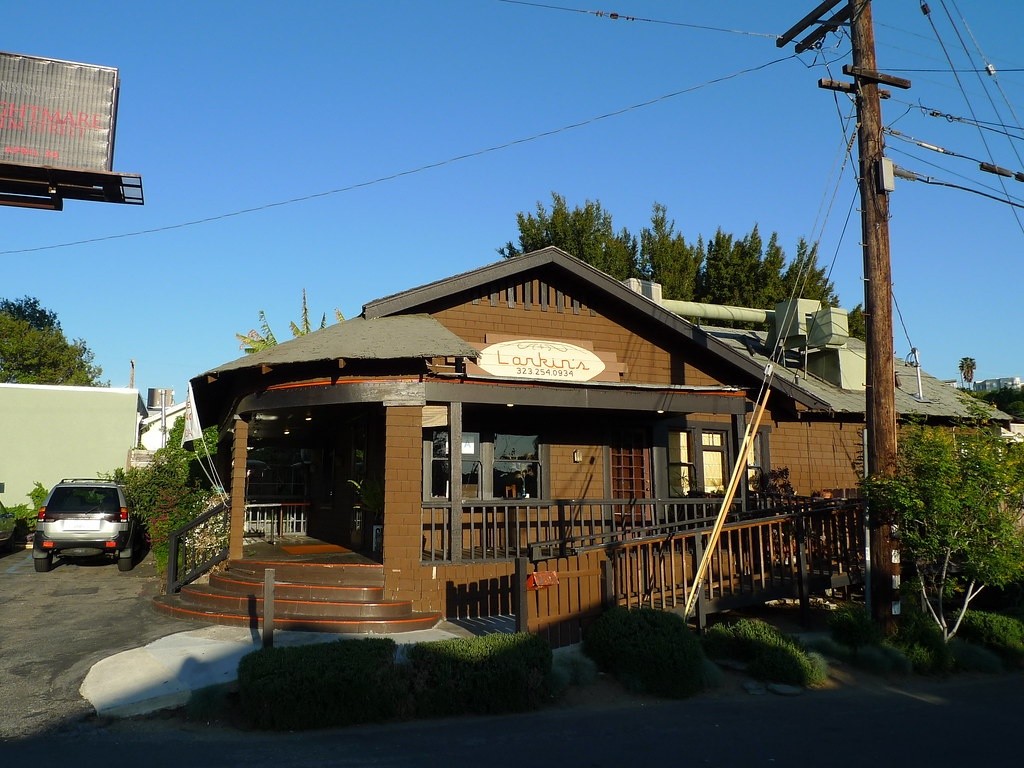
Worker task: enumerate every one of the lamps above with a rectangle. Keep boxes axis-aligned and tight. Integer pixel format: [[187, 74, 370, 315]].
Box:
[[730, 344, 756, 357]]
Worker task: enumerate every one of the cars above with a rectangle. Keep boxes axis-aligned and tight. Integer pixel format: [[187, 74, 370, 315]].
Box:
[[0, 501, 17, 555]]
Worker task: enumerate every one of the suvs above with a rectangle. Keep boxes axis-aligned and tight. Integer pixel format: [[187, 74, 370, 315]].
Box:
[[32, 478, 137, 573]]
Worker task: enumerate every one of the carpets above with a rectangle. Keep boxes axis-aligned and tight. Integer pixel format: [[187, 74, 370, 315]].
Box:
[[281, 544, 353, 555]]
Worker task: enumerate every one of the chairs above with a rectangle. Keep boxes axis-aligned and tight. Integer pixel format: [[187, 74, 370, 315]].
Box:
[[97, 494, 120, 513], [64, 496, 88, 512]]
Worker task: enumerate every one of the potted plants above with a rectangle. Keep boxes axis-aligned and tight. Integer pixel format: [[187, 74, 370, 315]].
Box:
[[345, 478, 385, 550]]
